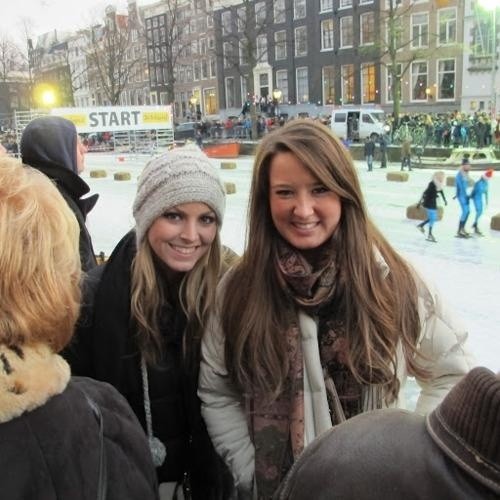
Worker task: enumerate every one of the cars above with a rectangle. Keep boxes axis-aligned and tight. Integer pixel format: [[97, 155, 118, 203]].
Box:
[[0, 119, 27, 134], [86, 106, 289, 139]]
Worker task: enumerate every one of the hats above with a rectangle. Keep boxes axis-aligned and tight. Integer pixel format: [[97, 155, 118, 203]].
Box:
[[133, 141, 226, 244], [485, 167, 493, 176]]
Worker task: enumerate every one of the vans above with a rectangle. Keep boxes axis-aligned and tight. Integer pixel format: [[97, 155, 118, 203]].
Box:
[[330, 108, 392, 143]]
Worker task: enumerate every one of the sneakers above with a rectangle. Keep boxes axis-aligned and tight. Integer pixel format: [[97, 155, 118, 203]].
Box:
[[474, 230, 484, 236], [454, 230, 474, 238], [426, 234, 438, 242], [416, 225, 425, 233]]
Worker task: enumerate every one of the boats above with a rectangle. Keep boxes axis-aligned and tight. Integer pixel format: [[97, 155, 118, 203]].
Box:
[[406, 145, 500, 171], [198, 138, 244, 159]]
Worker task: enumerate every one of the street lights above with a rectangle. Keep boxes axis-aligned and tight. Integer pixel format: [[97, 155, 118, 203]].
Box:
[[190, 94, 199, 136], [272, 88, 283, 116], [476, 0, 500, 105]]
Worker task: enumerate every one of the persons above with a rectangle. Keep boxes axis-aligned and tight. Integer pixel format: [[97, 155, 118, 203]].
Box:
[[0, 143, 161, 500], [192, 90, 286, 148], [338, 109, 500, 171], [270, 367, 500, 499], [80, 132, 111, 153], [455, 159, 474, 237], [21, 116, 102, 271], [416, 172, 447, 242], [197, 119, 470, 499], [470, 169, 493, 236], [57, 146, 243, 500]]
